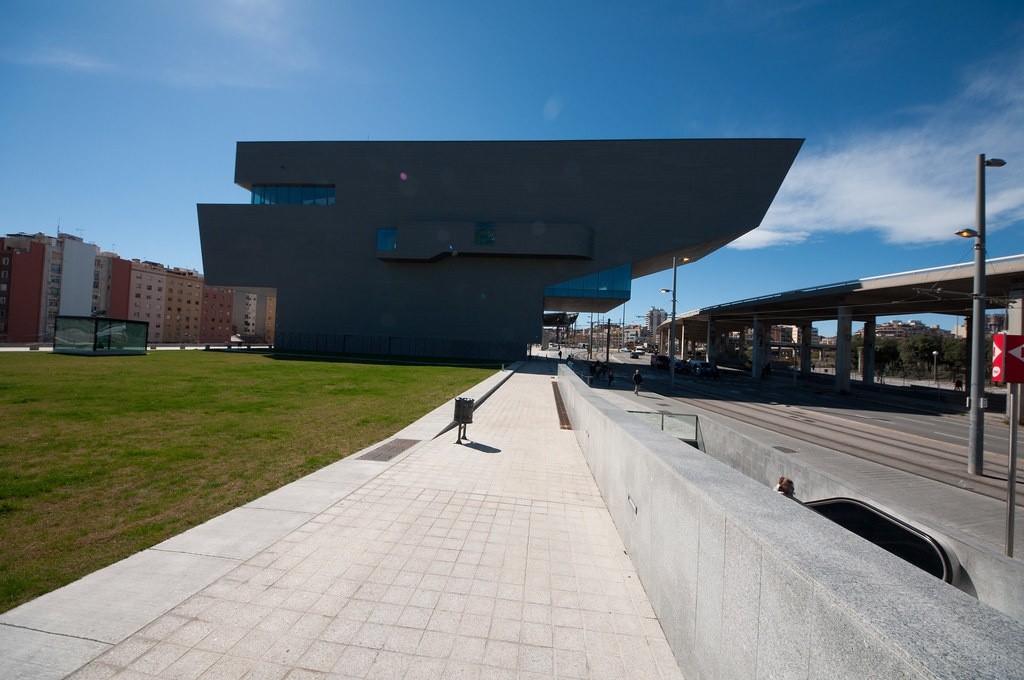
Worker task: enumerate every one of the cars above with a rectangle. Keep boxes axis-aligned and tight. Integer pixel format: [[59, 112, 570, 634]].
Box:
[[651, 355, 719, 377]]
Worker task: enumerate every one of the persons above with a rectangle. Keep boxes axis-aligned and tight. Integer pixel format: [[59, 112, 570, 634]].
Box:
[[633, 370, 643, 396], [589, 361, 615, 388]]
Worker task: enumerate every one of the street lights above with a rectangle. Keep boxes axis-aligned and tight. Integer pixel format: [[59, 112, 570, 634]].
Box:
[[660, 256, 690, 390], [954, 153, 1007, 475], [933, 351, 938, 383]]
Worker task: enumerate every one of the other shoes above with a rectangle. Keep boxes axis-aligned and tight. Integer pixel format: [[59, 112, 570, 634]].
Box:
[[635, 393, 640, 396]]
